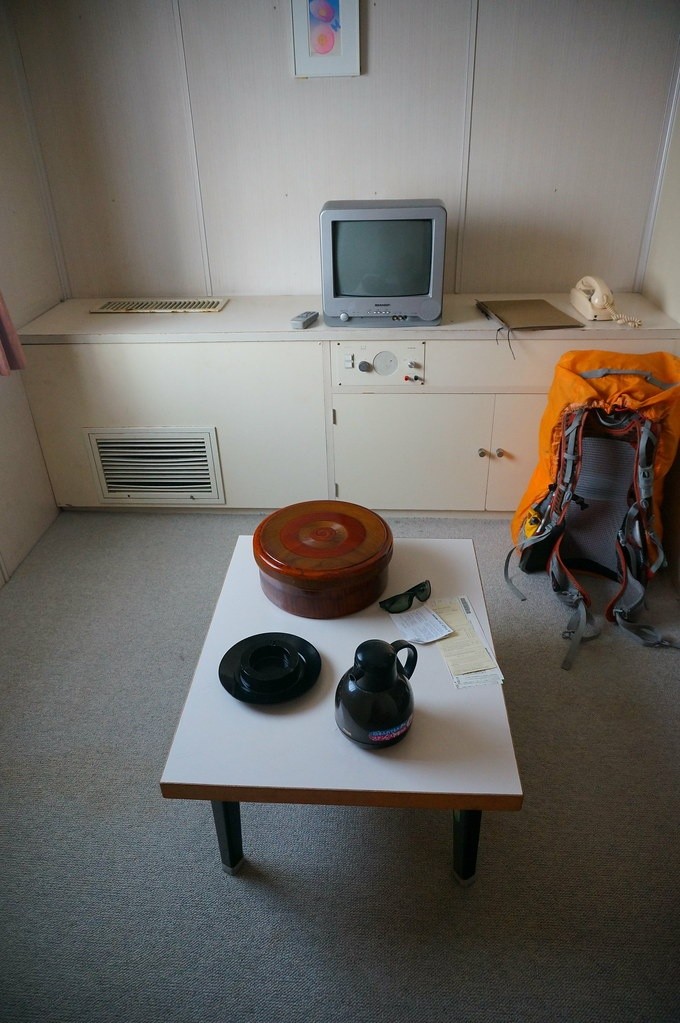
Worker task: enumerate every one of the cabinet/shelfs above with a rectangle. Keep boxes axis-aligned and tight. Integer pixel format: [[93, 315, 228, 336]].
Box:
[[13, 290, 680, 517]]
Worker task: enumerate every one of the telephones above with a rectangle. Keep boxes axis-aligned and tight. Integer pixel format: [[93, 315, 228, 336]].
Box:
[[569, 275, 616, 321]]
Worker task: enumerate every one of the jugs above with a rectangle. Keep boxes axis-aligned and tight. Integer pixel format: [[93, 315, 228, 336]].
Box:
[[334, 638, 419, 750]]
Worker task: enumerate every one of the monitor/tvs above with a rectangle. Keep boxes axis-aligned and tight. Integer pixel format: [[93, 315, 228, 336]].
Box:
[[318, 200, 447, 327]]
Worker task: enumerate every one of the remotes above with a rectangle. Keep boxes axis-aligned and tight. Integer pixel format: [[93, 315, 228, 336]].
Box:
[[290, 311, 319, 328]]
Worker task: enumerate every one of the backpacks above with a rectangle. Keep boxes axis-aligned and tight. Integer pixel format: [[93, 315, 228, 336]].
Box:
[[504, 350, 680, 671]]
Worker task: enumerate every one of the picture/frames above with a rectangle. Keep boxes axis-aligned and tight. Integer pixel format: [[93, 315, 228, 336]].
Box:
[[289, 0, 362, 78]]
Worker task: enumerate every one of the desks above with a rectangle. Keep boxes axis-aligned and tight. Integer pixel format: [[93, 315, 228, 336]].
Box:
[[160, 535, 524, 883]]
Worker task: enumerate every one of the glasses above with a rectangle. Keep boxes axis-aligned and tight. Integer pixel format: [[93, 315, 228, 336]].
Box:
[[379, 580, 431, 614]]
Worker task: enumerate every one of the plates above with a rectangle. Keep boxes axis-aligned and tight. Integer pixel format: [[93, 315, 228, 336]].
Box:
[[217, 631, 322, 706]]
